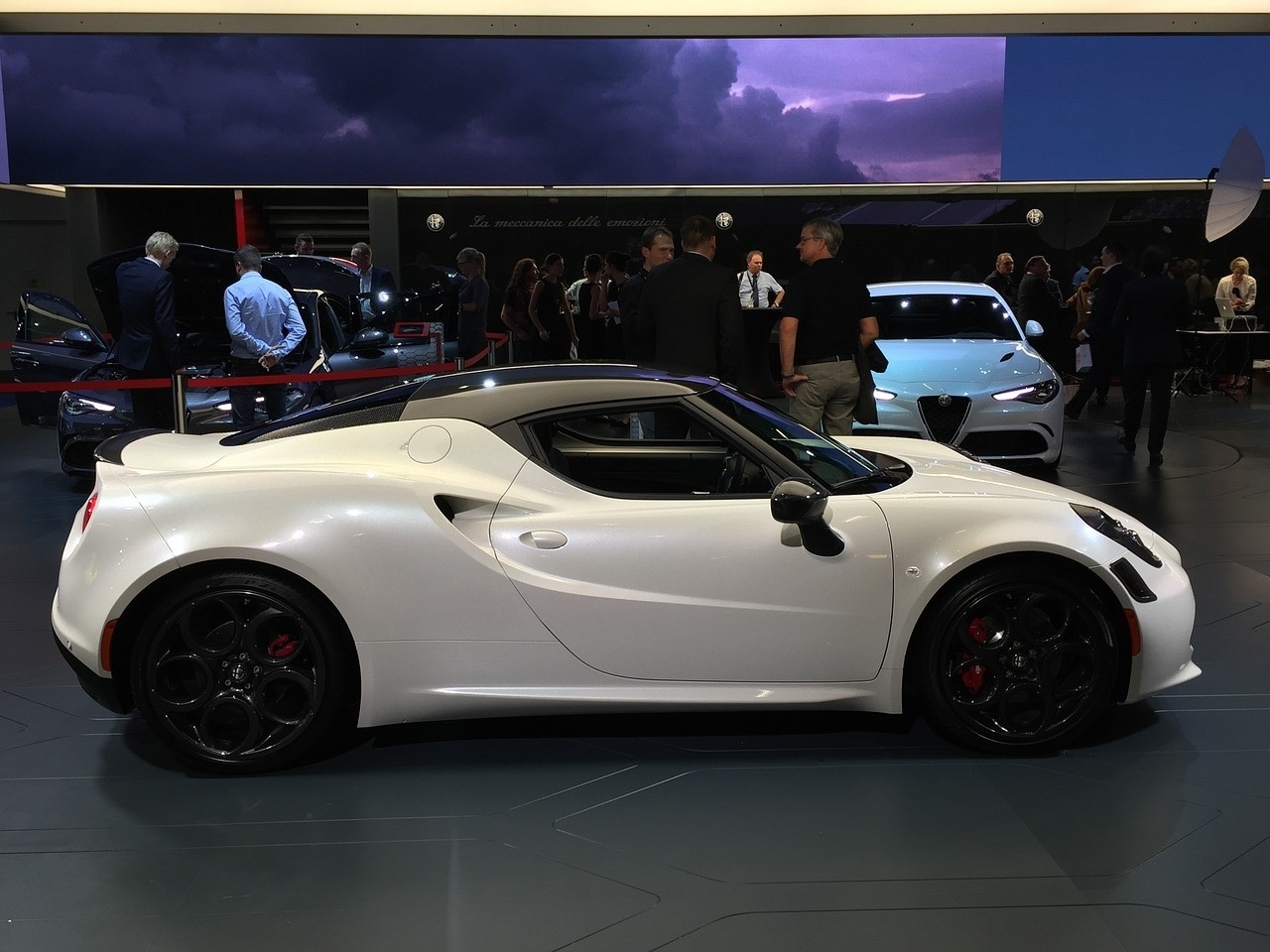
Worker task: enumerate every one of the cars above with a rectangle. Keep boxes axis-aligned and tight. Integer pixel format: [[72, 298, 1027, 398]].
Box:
[[820, 279, 1065, 478], [10, 242, 458, 478]]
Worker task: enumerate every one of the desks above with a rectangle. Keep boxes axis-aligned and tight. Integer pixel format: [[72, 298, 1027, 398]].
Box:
[[1171, 329, 1270, 402]]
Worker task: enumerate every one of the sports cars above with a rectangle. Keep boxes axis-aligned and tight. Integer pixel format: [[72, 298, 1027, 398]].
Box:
[[49, 360, 1205, 781]]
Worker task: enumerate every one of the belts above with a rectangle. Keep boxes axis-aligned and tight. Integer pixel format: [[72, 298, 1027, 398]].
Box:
[[794, 353, 852, 366]]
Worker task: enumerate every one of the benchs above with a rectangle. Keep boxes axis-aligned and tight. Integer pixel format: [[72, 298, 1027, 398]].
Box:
[[890, 313, 949, 340]]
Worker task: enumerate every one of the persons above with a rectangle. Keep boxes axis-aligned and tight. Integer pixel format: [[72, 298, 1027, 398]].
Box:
[[114, 216, 879, 438], [986, 241, 1256, 468]]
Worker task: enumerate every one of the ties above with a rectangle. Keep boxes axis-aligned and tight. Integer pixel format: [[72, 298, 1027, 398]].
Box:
[[752, 274, 760, 308]]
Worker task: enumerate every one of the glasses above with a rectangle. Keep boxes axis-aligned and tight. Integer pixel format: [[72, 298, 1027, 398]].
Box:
[[457, 261, 469, 265], [801, 236, 828, 243]]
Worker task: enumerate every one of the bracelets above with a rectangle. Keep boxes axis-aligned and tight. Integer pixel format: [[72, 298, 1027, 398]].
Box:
[[780, 371, 794, 379]]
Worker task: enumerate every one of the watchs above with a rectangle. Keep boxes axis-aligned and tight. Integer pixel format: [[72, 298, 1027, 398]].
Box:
[[773, 302, 779, 308]]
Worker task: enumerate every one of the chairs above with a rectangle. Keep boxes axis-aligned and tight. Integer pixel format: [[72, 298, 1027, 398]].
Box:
[[535, 420, 572, 479], [953, 300, 980, 332], [868, 302, 895, 338]]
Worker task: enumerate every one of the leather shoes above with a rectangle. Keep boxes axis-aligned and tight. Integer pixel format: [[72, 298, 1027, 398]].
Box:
[[1150, 452, 1164, 466], [1113, 417, 1125, 426], [1118, 426, 1136, 452]]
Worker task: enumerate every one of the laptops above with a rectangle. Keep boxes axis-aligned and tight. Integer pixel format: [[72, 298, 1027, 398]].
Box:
[[1215, 298, 1256, 320]]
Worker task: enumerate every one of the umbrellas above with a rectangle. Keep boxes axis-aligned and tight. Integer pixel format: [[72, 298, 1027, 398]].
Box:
[[1204, 125, 1264, 239]]
